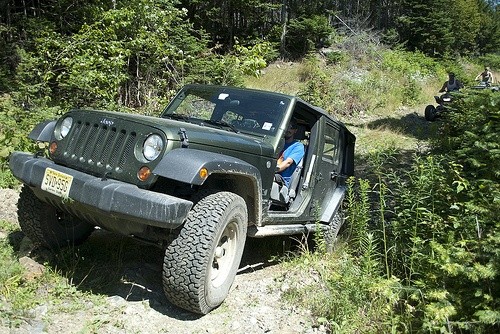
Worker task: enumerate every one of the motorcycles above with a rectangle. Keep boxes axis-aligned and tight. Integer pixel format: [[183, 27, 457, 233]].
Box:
[[425, 89, 459, 121], [471, 80, 498, 92]]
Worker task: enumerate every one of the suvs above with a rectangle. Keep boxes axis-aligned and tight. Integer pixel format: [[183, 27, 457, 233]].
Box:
[[11, 82, 359, 319]]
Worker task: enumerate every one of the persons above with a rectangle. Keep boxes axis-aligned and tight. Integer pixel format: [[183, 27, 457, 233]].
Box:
[[436, 73, 465, 92], [274, 118, 305, 189], [473, 66, 493, 86]]
